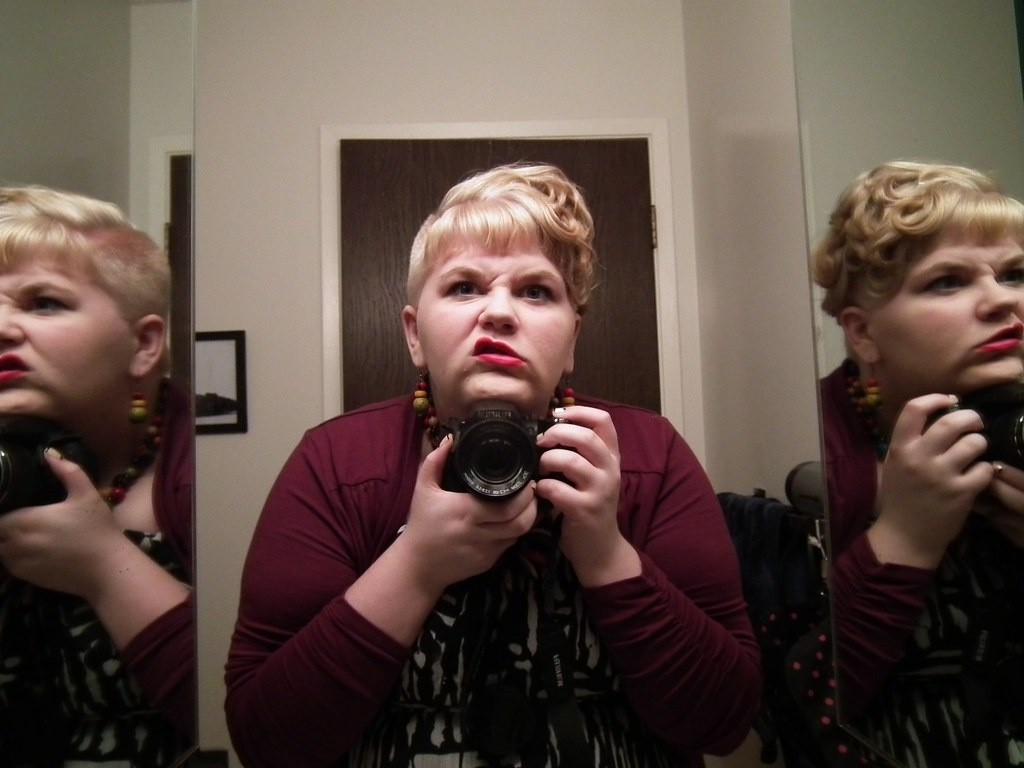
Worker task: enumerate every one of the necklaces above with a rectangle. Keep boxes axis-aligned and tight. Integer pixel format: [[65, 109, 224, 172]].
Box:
[[842, 352, 891, 463], [100, 442, 157, 510], [419, 383, 562, 463]]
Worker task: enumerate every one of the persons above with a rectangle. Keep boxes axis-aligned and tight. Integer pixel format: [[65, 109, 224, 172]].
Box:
[[1, 185, 198, 768], [223, 162, 761, 768], [817, 159, 1024, 768]]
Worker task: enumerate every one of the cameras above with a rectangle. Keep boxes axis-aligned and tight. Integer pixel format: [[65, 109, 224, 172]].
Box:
[[935, 381, 1024, 499], [435, 399, 570, 502], [0, 412, 95, 515]]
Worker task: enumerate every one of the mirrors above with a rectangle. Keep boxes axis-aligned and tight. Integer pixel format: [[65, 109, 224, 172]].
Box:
[[0, 0, 1024, 768]]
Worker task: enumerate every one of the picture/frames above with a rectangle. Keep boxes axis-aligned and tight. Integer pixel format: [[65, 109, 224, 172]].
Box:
[[196, 329, 248, 435]]
